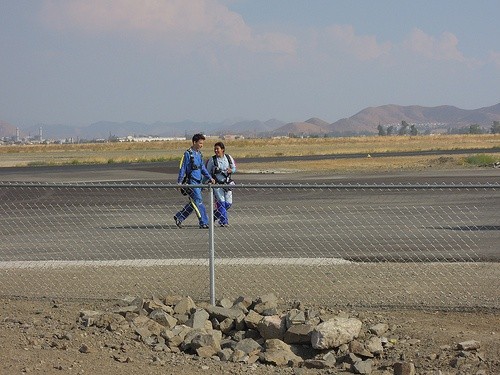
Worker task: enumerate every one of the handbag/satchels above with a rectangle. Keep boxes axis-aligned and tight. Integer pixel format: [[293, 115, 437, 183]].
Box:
[[181, 177, 190, 196]]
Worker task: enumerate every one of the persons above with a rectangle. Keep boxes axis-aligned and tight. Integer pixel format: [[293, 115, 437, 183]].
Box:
[[204, 141, 236, 228], [173, 133, 215, 228]]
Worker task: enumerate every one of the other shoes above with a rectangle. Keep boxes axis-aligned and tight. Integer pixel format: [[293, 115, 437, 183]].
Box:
[[174, 216, 182, 228], [199, 224, 210, 229], [220, 223, 227, 227]]
